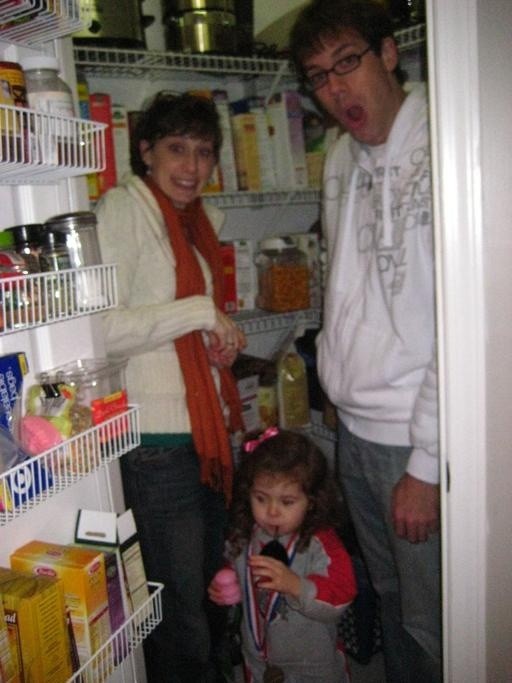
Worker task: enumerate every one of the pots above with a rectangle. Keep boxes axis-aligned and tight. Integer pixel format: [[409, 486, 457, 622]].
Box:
[[165, 9, 244, 61]]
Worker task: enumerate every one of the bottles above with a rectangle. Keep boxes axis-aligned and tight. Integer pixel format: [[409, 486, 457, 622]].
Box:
[[260, 231, 314, 312], [253, 542, 289, 592], [0, 61, 109, 309]]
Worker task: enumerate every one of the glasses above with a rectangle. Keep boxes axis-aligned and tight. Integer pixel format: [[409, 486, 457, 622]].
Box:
[[299, 40, 373, 94]]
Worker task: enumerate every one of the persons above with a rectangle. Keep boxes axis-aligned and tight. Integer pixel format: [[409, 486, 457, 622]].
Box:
[[90, 86, 246, 680], [285, 0, 443, 683], [204, 427, 363, 682]]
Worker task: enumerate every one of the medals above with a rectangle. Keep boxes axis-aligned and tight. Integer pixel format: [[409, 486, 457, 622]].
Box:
[[263, 665, 285, 683]]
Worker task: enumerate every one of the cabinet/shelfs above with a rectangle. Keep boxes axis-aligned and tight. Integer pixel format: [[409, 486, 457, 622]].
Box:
[[1, 0, 427, 683]]
